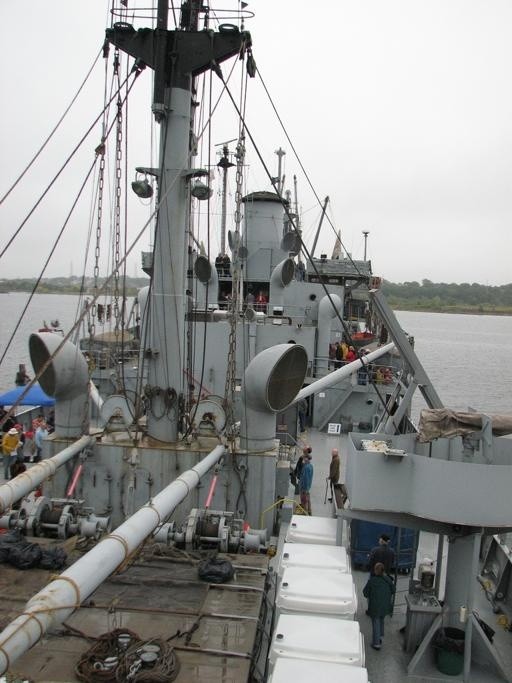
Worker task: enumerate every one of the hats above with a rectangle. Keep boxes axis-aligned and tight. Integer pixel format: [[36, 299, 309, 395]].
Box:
[[332, 340, 369, 354], [7, 423, 24, 437], [378, 533, 391, 542]]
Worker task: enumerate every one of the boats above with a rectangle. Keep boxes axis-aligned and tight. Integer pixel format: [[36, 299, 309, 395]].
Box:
[[0, 0, 512, 683]]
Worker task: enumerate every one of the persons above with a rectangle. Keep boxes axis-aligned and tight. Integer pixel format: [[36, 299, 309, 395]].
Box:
[[362, 562, 396, 650], [1, 404, 55, 480], [327, 324, 392, 386], [325, 447, 340, 504], [294, 445, 313, 507], [299, 453, 312, 516], [245, 289, 256, 307], [222, 253, 231, 276], [255, 289, 267, 314], [214, 252, 222, 277], [365, 533, 396, 617]]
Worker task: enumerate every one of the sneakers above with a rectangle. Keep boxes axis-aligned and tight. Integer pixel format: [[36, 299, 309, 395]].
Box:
[[370, 637, 384, 651]]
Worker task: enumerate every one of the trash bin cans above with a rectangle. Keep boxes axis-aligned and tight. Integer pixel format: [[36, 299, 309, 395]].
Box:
[[432, 627, 465, 674]]
